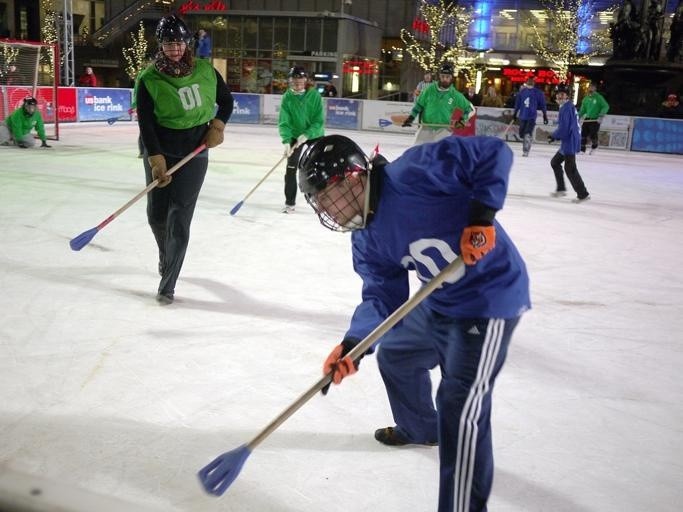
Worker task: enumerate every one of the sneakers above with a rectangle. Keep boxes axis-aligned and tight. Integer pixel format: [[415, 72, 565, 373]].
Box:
[[158, 291, 174, 303], [573, 195, 590, 203], [551, 190, 566, 198], [282, 205, 294, 214], [375, 426, 438, 447]]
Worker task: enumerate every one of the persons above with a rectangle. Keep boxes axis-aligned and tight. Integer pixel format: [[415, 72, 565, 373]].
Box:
[[297, 133, 532, 512], [401, 65, 478, 144], [78, 66, 97, 87], [546, 85, 592, 203], [277, 65, 326, 214], [658, 88, 682, 119], [240, 64, 337, 98], [195, 29, 211, 59], [509, 79, 549, 159], [481, 82, 574, 110], [577, 85, 610, 156], [463, 84, 479, 106], [412, 70, 434, 101], [1, 96, 50, 149], [0, 62, 25, 86], [132, 14, 234, 305]]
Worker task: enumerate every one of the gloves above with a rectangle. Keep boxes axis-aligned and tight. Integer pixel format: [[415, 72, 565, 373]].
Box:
[[147, 155, 172, 187], [402, 116, 414, 126], [201, 120, 225, 147], [455, 121, 464, 130], [283, 143, 291, 158], [322, 337, 361, 395], [461, 225, 496, 265]]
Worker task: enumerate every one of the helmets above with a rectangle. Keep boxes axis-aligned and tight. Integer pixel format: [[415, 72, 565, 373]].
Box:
[[153, 13, 193, 75], [438, 65, 453, 87], [554, 84, 570, 103], [287, 66, 308, 91], [295, 134, 372, 233], [24, 96, 37, 116]]
